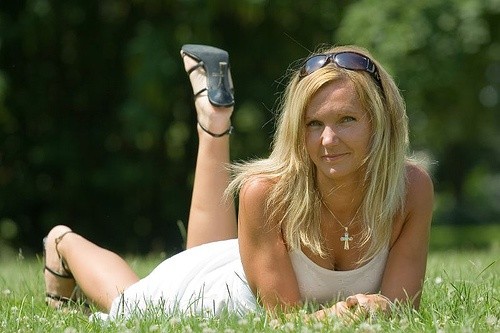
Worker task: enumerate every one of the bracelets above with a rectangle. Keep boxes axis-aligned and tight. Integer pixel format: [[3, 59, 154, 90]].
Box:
[[375, 294, 394, 307]]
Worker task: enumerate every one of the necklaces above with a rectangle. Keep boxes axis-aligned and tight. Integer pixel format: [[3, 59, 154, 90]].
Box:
[[316, 186, 364, 251]]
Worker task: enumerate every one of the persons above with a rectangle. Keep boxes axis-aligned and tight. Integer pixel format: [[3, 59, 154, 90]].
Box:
[[43, 44, 434, 321]]
